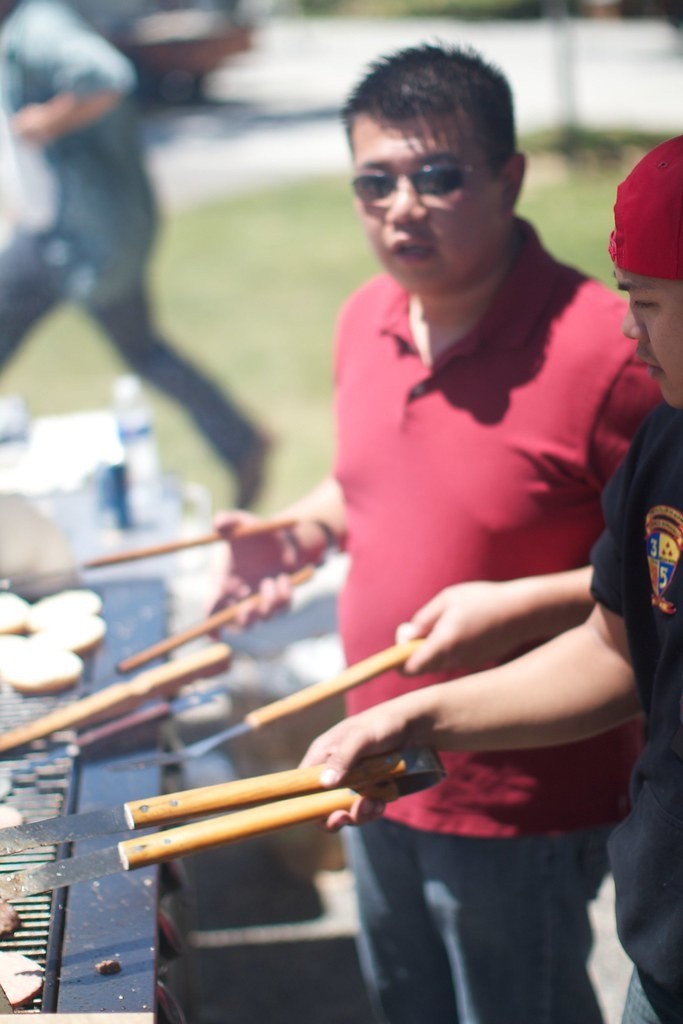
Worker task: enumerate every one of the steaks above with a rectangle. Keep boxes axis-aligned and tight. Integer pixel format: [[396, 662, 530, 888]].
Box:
[[0, 900, 19, 936]]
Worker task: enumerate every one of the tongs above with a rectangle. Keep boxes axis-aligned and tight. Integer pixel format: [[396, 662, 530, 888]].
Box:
[[0, 519, 332, 709], [0, 746, 446, 900]]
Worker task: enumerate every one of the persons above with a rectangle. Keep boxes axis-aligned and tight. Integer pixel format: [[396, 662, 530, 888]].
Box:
[[0, 0, 278, 513], [297, 136, 683, 1024], [205, 40, 663, 1024]]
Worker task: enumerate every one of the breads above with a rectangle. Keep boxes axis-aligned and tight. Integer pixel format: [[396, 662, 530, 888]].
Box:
[[0, 588, 107, 695]]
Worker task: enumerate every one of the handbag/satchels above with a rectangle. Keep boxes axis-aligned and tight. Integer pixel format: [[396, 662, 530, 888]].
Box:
[[10, 72, 154, 311]]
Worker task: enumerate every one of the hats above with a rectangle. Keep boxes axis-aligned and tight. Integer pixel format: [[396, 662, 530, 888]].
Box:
[[608, 135, 683, 280]]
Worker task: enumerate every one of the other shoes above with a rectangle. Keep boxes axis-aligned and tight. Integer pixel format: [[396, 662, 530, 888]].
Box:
[[229, 435, 267, 509]]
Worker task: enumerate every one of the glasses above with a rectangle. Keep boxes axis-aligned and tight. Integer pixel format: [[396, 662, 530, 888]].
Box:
[[350, 168, 465, 194]]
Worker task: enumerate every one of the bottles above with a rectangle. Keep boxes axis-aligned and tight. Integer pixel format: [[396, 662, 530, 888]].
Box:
[[114, 378, 158, 500]]
